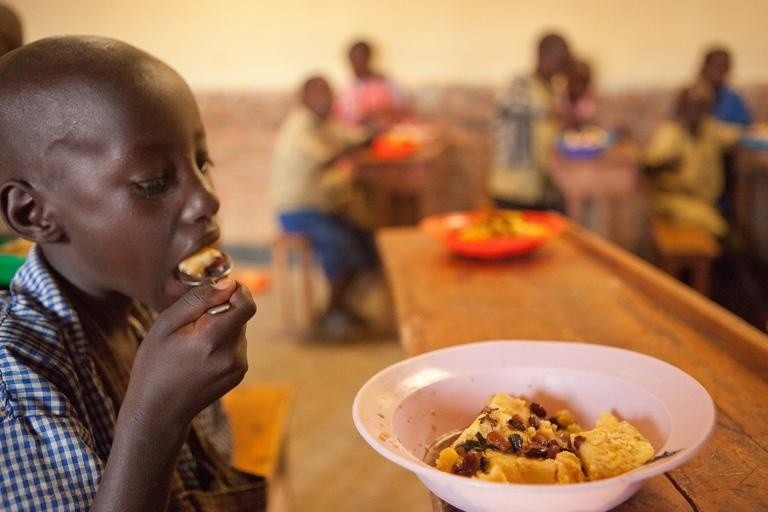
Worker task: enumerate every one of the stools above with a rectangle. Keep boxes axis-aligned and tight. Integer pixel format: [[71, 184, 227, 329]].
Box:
[[645, 216, 719, 294], [271, 232, 321, 337]]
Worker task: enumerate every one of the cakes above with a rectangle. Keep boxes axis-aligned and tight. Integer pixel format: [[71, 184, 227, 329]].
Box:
[[436, 394, 655, 484], [177, 246, 223, 277]]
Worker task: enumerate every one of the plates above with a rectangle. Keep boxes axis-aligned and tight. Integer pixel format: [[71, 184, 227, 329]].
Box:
[[416, 204, 566, 261]]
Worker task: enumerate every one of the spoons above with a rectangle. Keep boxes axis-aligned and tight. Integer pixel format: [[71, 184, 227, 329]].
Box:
[[174, 250, 236, 320]]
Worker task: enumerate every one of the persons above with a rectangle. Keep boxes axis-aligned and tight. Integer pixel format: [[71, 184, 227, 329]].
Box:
[[1, 32, 266, 511], [2, 7, 36, 285], [635, 48, 754, 296], [339, 41, 391, 122], [488, 34, 598, 214], [271, 75, 377, 343]]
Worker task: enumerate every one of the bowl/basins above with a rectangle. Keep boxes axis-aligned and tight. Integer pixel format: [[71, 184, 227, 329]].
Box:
[[350, 335, 719, 510]]
